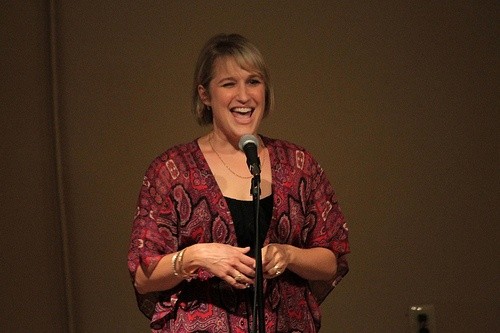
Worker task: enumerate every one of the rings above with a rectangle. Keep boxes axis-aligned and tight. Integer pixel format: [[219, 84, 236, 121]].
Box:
[[274, 264, 281, 276], [235, 270, 244, 280]]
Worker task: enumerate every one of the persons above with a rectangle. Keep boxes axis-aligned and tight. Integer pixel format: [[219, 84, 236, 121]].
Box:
[[128, 33, 349, 333]]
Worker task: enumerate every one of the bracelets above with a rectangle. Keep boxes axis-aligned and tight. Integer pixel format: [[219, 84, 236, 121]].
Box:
[[180, 247, 202, 278], [172, 250, 195, 282]]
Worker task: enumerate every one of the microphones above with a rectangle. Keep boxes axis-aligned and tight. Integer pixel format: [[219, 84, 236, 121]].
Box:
[[238, 135, 260, 179]]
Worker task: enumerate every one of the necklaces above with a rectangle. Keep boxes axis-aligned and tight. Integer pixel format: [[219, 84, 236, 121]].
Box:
[[207, 133, 266, 178]]
